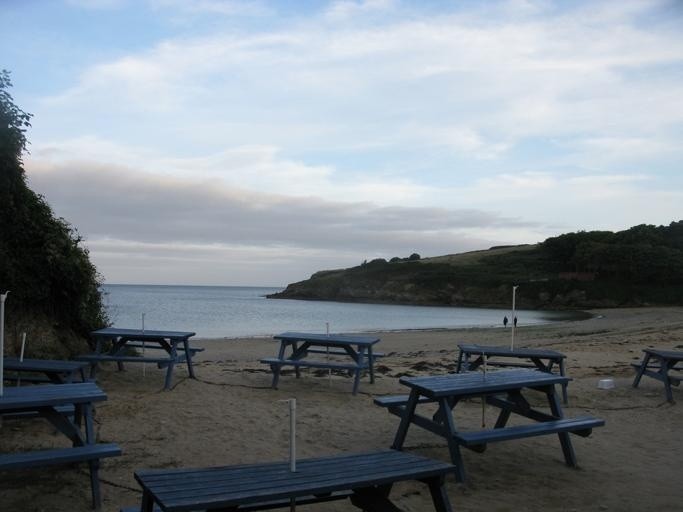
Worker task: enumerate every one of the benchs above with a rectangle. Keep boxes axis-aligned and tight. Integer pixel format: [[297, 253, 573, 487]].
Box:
[[259, 324, 680, 481], [0, 325, 204, 511], [132, 446, 461, 512]]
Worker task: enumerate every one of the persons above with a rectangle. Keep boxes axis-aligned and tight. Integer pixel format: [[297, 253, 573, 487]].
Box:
[[503, 315, 508, 328], [513, 316, 517, 328]]
